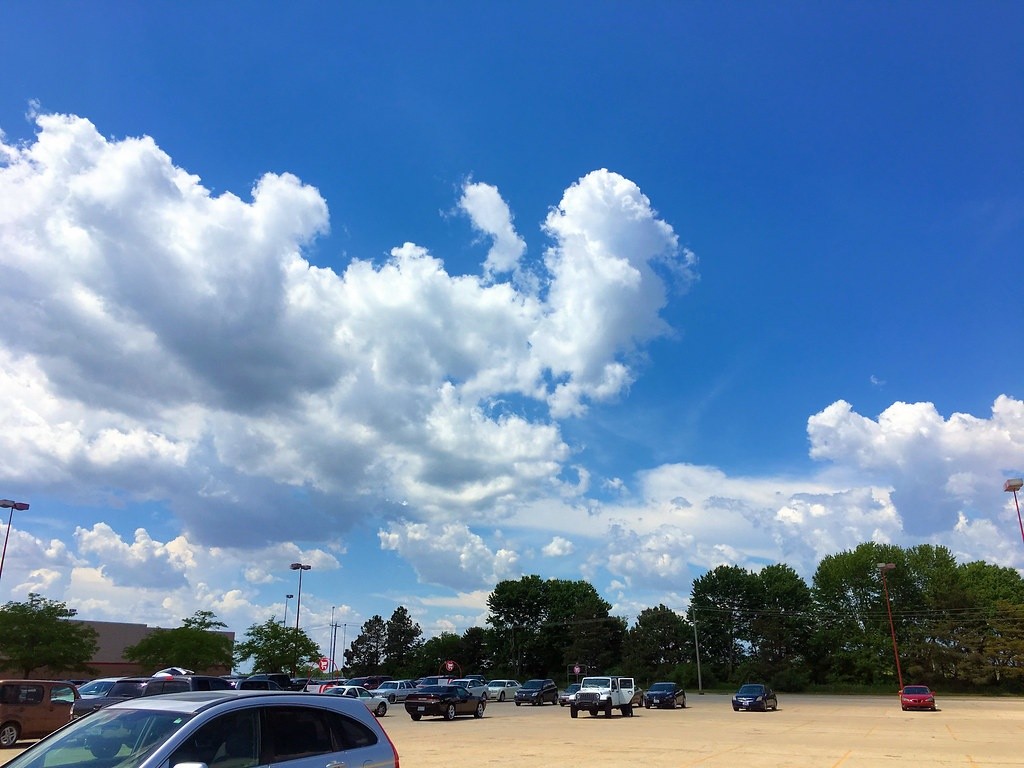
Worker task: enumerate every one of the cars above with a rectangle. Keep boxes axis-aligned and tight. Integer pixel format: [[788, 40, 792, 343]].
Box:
[[630, 685, 643, 707], [644, 682, 686, 709], [732, 683, 778, 712], [449, 678, 489, 700], [405, 685, 486, 721], [899, 685, 936, 711], [0, 667, 425, 768], [485, 680, 522, 702], [558, 683, 582, 706], [414, 674, 458, 690]]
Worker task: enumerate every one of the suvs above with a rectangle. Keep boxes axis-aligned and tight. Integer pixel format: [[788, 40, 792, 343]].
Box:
[[568, 676, 634, 719], [513, 679, 559, 706], [465, 674, 486, 685]]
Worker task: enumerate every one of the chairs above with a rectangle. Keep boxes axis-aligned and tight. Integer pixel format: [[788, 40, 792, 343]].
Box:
[[210, 717, 275, 768], [22, 692, 36, 704], [147, 716, 201, 768]]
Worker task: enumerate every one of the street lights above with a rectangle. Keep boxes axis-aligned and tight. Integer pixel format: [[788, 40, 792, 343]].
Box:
[[1003, 478, 1024, 541], [289, 563, 311, 679], [877, 562, 904, 690], [0, 499, 30, 579], [283, 595, 294, 628]]
